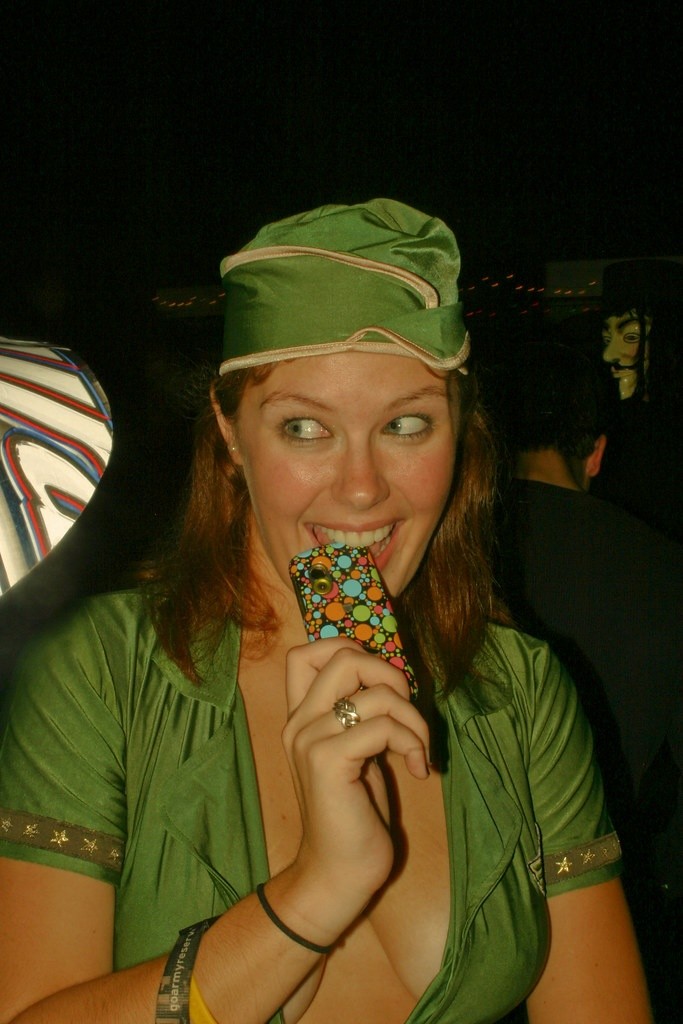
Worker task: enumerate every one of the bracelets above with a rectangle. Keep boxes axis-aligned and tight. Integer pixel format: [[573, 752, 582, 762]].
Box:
[[156, 920, 212, 1024], [256, 884, 332, 954]]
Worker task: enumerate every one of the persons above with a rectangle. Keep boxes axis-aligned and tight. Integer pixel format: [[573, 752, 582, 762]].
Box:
[[481, 341, 682, 909], [0, 198, 653, 1024]]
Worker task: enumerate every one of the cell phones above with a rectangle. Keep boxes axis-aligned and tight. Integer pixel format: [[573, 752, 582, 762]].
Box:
[[287, 542, 420, 703]]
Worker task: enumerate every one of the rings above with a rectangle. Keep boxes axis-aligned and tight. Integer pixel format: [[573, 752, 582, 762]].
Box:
[[332, 698, 360, 728]]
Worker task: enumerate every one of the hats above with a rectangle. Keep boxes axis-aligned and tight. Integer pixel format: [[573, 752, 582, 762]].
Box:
[[219, 199, 471, 376]]
